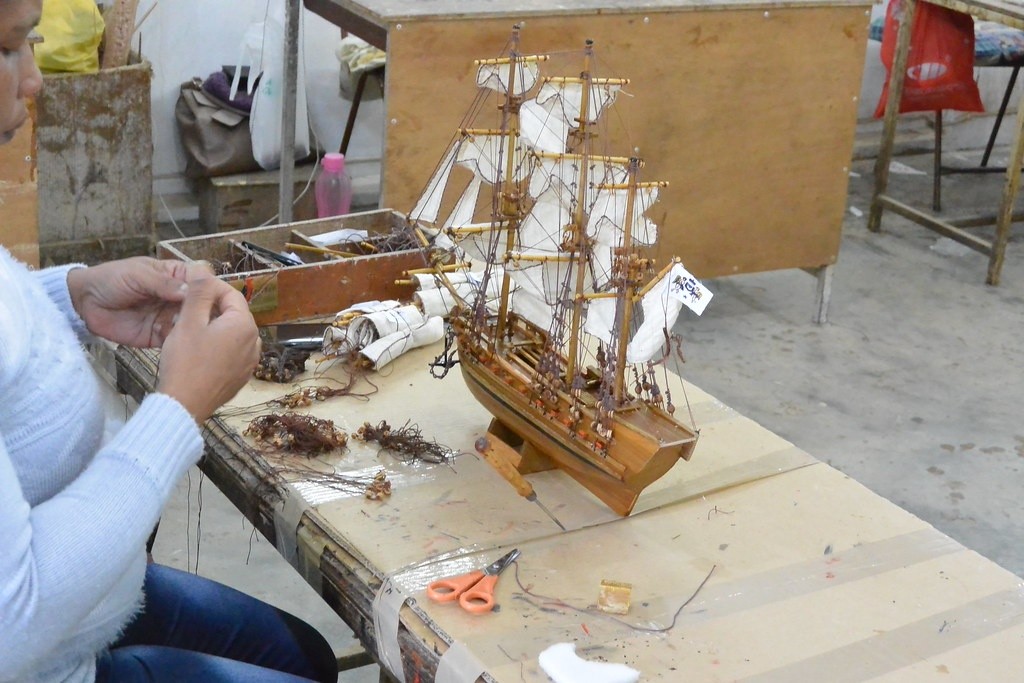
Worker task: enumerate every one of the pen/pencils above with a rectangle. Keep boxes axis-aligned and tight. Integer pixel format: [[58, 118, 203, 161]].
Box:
[[242, 240, 302, 266], [278, 338, 325, 350]]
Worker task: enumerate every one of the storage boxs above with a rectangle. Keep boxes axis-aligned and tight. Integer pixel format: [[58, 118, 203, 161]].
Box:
[[154, 209, 456, 347]]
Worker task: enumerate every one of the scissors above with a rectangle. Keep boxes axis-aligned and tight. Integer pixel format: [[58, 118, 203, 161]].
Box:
[[424, 545, 523, 617]]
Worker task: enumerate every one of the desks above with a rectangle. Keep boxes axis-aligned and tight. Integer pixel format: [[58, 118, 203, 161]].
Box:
[[278, 0, 877, 327], [864, 0, 1024, 287], [82, 208, 1024, 682]]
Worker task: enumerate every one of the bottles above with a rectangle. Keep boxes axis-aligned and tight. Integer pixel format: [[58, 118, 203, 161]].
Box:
[[315, 153, 352, 219]]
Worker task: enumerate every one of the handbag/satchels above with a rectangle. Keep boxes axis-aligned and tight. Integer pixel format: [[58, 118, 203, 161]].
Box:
[[175, 66, 326, 178], [229, 0, 310, 170], [34, 0, 105, 72], [874, 0, 984, 119]]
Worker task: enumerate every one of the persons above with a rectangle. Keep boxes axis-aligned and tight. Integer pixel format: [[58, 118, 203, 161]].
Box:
[[1, 0, 338, 683]]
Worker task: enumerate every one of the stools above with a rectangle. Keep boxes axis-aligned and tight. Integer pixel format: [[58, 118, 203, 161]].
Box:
[[869, 0, 1024, 213]]
